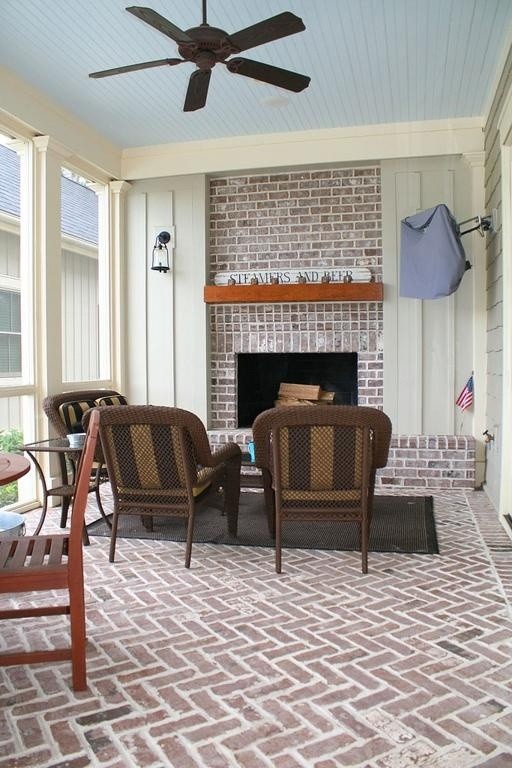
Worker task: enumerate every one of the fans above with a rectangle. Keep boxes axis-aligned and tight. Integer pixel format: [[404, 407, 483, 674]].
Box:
[[90, 0, 311, 112]]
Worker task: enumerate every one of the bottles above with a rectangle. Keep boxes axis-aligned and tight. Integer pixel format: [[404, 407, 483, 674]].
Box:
[[248, 440, 256, 462]]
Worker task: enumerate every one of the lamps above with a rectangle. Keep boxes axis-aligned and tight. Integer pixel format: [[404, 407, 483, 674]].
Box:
[[151, 231, 170, 272]]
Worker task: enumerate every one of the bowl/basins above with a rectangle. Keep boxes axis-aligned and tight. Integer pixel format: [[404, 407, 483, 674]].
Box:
[[65, 432, 86, 443], [69, 444, 84, 449]]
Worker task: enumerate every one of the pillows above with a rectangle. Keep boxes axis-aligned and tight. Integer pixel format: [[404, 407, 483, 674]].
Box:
[[95, 395, 128, 406], [58, 400, 95, 432]]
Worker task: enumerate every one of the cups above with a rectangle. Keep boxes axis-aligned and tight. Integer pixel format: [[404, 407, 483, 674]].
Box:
[[227, 275, 353, 286]]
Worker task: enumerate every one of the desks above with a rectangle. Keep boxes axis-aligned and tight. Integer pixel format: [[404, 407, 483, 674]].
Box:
[[211, 450, 263, 487], [0, 452, 30, 486], [20, 438, 114, 544]]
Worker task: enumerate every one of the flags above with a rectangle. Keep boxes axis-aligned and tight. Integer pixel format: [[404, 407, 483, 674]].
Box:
[[454, 376, 473, 413]]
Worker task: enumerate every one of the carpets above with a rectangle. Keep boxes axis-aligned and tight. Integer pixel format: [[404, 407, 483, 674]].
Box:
[[85, 491, 440, 555]]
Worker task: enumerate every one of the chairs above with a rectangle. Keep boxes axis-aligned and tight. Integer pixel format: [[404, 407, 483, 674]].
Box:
[[42, 390, 129, 491], [82, 405, 242, 568], [0, 410, 100, 690], [253, 405, 392, 574]]
